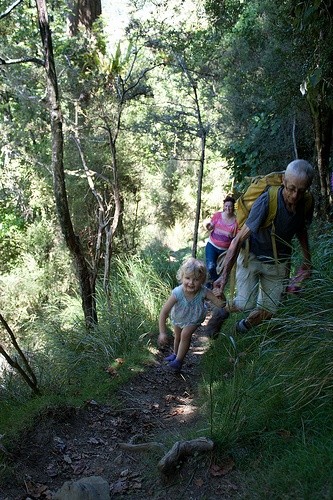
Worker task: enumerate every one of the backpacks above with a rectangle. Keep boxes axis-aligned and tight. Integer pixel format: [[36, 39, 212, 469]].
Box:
[[235, 170, 313, 230]]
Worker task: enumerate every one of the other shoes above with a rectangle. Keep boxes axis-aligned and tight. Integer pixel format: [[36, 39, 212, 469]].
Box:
[[207, 307, 229, 340], [236, 320, 248, 334], [167, 360, 182, 369], [164, 354, 177, 363]]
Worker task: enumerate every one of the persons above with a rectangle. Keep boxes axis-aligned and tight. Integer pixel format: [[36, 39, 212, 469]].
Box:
[[205, 160, 315, 340], [158, 258, 227, 370]]
